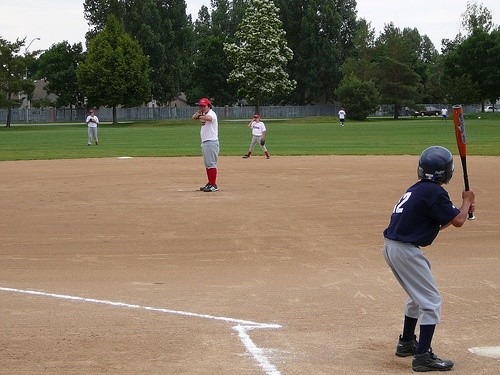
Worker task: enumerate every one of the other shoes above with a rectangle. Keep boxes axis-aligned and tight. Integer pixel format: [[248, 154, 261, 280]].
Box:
[[200, 183, 210, 191], [342, 125, 344, 126], [95, 142, 99, 144], [340, 124, 342, 125], [242, 155, 250, 159], [267, 155, 270, 159], [88, 144, 90, 146]]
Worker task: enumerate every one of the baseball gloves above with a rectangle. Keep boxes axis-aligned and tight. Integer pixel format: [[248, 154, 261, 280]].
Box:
[[261, 139, 265, 146], [192, 110, 203, 120]]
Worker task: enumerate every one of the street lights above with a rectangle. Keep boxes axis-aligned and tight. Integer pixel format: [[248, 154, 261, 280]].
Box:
[[24, 37, 40, 108]]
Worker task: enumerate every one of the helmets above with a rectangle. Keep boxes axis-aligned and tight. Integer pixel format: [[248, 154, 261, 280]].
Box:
[[417, 146, 455, 184]]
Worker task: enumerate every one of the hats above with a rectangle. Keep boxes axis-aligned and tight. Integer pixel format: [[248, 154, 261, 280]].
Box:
[[198, 98, 212, 106], [90, 110, 94, 113], [253, 114, 260, 118]]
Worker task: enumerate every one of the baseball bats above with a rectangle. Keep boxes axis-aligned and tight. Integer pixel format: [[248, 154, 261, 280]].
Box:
[[452, 105, 476, 220]]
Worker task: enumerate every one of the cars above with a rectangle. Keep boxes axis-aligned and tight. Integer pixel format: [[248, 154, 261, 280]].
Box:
[[476, 106, 496, 113], [415, 107, 442, 116]]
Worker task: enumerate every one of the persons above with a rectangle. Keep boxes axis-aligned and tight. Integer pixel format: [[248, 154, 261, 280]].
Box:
[[86, 109, 100, 146], [405, 106, 409, 116], [383, 146, 476, 372], [192, 98, 218, 191], [242, 114, 270, 159], [441, 107, 447, 121], [338, 108, 346, 126]]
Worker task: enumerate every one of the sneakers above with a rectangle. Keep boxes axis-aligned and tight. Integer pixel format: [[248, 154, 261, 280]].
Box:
[[412, 347, 454, 372], [395, 334, 420, 357], [204, 184, 218, 192]]
